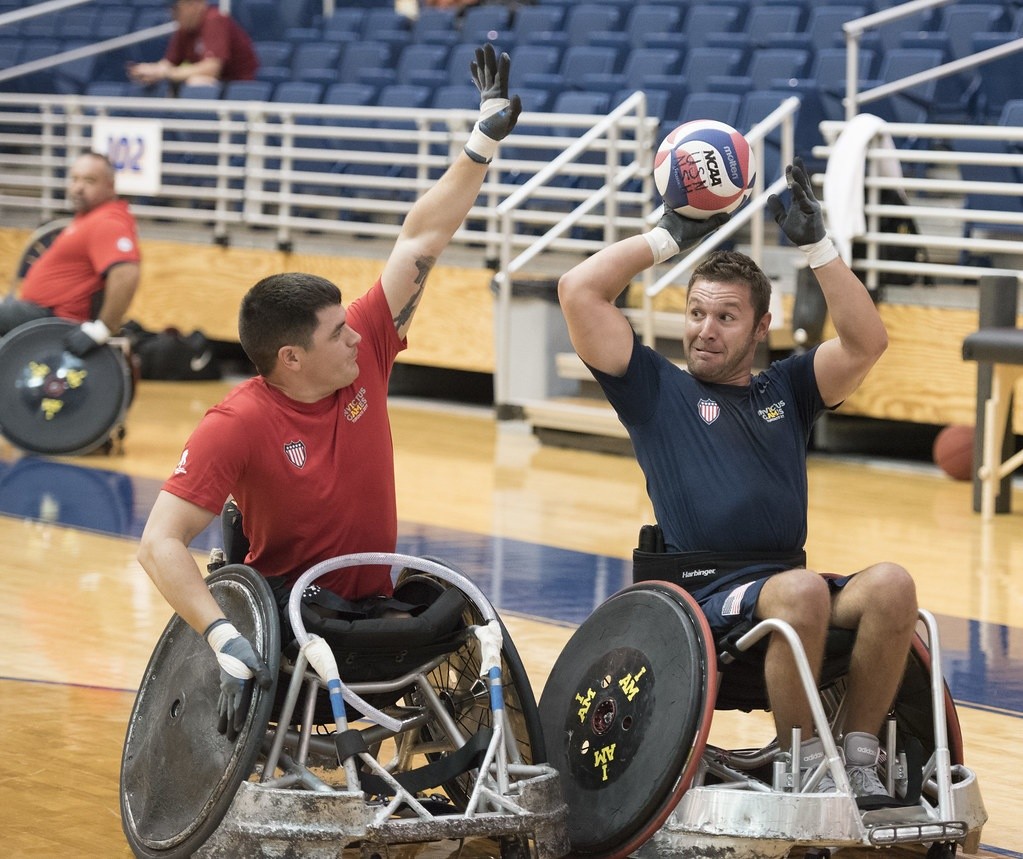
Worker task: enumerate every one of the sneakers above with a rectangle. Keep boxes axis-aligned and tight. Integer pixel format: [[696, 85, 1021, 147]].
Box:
[[836, 730, 890, 796], [779, 737, 836, 794]]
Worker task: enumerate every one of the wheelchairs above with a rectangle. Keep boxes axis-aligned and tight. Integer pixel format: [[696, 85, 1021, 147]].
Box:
[[118, 498, 548, 859], [0, 317, 138, 452], [538, 523, 988, 859]]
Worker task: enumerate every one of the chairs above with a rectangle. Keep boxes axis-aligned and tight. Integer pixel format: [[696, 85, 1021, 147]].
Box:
[[0, 0, 1023, 285]]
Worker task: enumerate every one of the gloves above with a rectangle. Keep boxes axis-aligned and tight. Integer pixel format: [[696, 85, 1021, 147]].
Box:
[[642, 193, 731, 265], [61, 318, 112, 359], [201, 620, 272, 742], [768, 157, 838, 268], [462, 41, 521, 162]]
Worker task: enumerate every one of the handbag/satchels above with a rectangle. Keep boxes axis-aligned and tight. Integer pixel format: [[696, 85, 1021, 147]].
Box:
[[139, 327, 220, 381]]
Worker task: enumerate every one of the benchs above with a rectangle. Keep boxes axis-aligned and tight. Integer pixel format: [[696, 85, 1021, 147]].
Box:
[[961, 327, 1023, 522]]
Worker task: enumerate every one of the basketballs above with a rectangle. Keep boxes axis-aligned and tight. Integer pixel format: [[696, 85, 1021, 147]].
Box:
[[653, 118, 758, 222], [934, 424, 976, 482]]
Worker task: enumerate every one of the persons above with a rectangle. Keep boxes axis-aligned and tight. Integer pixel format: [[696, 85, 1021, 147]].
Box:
[[137, 42, 522, 741], [125, 0, 261, 86], [557, 156, 918, 795], [0, 151, 142, 358]]
